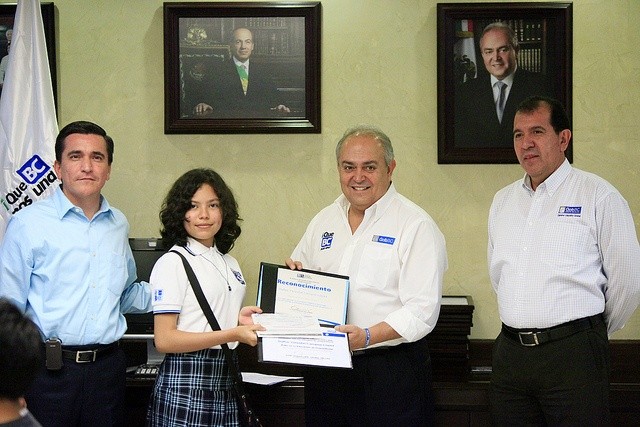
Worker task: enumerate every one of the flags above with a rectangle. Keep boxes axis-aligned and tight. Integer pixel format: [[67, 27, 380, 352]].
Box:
[[0, 0, 66, 265], [452, 16, 478, 82]]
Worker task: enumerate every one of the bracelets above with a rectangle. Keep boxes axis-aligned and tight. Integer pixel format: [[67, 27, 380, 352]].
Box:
[[364, 328, 369, 347]]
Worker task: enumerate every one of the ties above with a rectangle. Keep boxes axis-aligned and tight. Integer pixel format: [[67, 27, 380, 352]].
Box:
[[241, 65, 248, 93], [495, 82, 507, 124]]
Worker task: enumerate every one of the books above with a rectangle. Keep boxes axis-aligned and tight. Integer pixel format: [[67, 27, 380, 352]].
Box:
[[481, 18, 545, 72], [180, 18, 305, 56]]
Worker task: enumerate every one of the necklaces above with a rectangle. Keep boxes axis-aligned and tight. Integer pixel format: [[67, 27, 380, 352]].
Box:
[[201, 254, 231, 291]]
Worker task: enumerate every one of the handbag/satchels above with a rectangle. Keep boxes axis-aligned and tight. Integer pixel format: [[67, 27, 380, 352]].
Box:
[[233, 379, 263, 427]]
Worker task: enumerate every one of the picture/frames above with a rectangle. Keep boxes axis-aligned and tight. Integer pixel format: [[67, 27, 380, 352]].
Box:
[[436, 2, 574, 166], [0, 2, 58, 125], [162, 2, 322, 136]]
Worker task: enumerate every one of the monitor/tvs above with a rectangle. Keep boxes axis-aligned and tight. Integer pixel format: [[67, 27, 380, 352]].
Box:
[[119, 237, 167, 365]]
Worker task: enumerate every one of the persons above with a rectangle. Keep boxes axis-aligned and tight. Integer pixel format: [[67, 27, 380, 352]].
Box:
[[0, 121, 154, 427], [190, 26, 290, 113], [0, 29, 13, 85], [446, 21, 557, 155], [487, 95, 640, 427], [148, 168, 266, 427], [285, 126, 448, 427]]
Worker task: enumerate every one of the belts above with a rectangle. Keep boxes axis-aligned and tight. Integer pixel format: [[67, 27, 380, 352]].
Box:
[[502, 314, 604, 348], [351, 346, 389, 356], [61, 342, 120, 363]]
